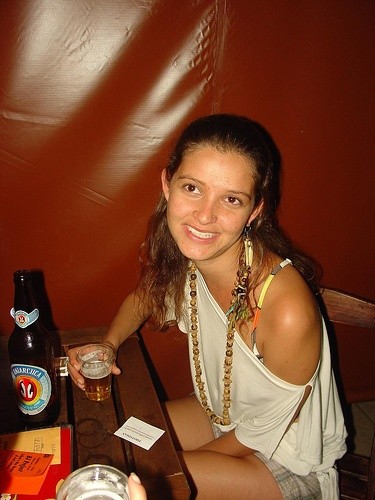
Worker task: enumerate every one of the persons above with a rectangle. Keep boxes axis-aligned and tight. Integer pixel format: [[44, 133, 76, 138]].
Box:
[[68, 114, 349, 500]]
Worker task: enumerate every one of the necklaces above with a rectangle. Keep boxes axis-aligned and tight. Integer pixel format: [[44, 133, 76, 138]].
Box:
[[189, 250, 245, 425]]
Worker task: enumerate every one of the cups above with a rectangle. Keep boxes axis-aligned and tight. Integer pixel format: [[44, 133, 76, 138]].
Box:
[[56, 463, 129, 500], [77, 343, 116, 402]]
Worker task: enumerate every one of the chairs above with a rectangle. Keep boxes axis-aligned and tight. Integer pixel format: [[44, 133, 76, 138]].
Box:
[[321, 288, 375, 500]]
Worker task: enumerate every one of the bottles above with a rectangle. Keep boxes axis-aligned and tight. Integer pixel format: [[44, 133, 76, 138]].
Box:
[[7, 269, 61, 428]]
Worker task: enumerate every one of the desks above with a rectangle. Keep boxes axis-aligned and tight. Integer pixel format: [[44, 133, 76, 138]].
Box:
[[52, 338, 194, 500]]
